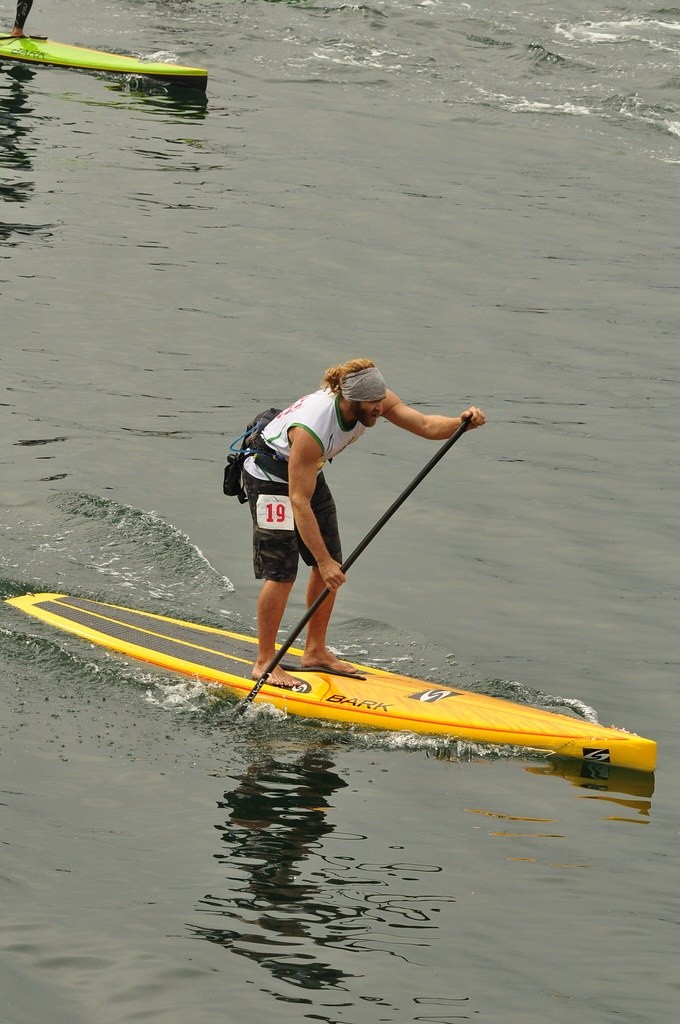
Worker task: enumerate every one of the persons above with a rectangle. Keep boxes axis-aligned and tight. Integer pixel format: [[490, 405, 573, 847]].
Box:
[[242, 359, 486, 688]]
